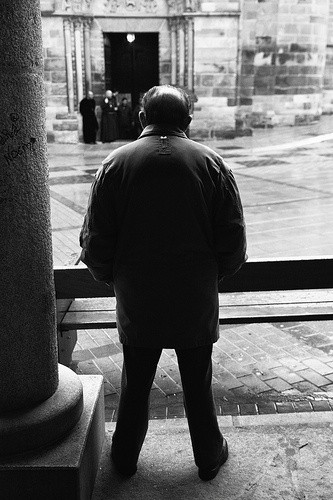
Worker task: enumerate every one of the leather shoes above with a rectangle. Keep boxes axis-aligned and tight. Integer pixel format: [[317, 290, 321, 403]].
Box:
[[111, 443, 137, 474], [197, 437, 229, 481]]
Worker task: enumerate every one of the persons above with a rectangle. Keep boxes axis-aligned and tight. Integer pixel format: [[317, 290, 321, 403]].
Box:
[[76, 84, 248, 481], [133, 99, 147, 137], [79, 90, 99, 144], [116, 97, 133, 139], [101, 89, 117, 143]]
